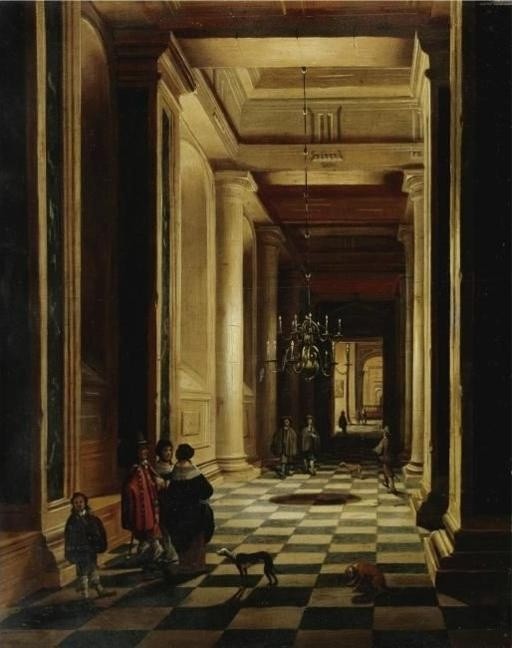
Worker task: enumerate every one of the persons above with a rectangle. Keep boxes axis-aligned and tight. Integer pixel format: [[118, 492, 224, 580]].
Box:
[[373, 425, 397, 495], [167, 442, 215, 576], [120, 433, 167, 569], [299, 414, 321, 476], [63, 492, 117, 604], [151, 438, 179, 564], [271, 415, 298, 481], [339, 410, 348, 433]]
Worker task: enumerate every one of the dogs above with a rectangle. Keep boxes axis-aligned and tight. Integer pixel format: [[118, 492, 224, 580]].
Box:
[[214, 546, 279, 586]]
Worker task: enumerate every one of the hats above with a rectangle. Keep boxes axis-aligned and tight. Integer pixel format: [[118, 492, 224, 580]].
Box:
[[385, 425, 390, 433], [175, 444, 194, 459], [282, 416, 293, 422]]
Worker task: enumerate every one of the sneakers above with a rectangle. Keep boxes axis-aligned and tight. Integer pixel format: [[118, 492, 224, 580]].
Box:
[[100, 589, 116, 597], [304, 467, 316, 475], [278, 470, 296, 477], [384, 482, 396, 493]]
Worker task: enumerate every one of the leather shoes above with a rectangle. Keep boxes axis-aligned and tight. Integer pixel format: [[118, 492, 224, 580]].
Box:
[[192, 566, 214, 576]]
